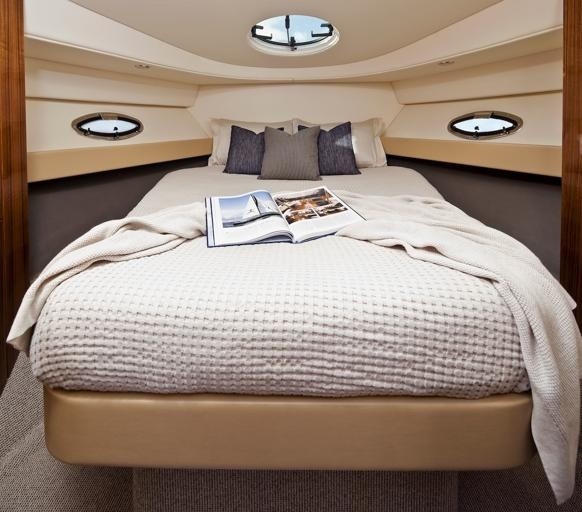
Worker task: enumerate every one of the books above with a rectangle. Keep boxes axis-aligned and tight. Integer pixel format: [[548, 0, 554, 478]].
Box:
[[206, 186, 366, 245]]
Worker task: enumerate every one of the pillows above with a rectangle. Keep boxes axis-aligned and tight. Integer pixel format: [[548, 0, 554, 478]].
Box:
[[292, 116, 388, 169], [298, 120, 361, 175], [256, 125, 323, 180], [222, 124, 284, 176], [207, 116, 292, 168]]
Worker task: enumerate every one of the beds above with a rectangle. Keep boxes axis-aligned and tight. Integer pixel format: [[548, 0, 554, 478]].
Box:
[[30, 117, 582, 473]]
